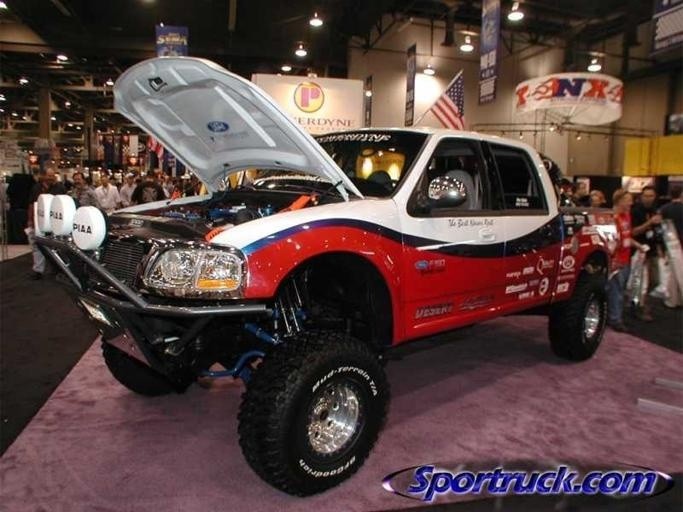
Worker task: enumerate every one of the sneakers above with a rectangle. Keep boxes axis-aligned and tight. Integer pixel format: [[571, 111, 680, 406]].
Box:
[[26, 271, 42, 279], [650, 288, 677, 308], [613, 323, 629, 332], [637, 312, 655, 322]]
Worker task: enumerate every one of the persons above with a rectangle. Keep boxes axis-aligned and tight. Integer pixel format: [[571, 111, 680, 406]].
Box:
[[558, 178, 683, 333], [6, 166, 197, 280]]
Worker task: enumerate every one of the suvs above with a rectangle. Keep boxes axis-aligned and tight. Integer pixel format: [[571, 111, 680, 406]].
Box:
[[27, 56, 620, 499]]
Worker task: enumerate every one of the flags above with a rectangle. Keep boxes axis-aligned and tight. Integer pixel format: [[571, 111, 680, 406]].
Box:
[[430, 70, 464, 130]]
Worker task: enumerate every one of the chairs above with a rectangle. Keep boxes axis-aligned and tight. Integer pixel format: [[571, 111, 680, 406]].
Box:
[[429, 169, 475, 213], [368, 171, 391, 190]]
